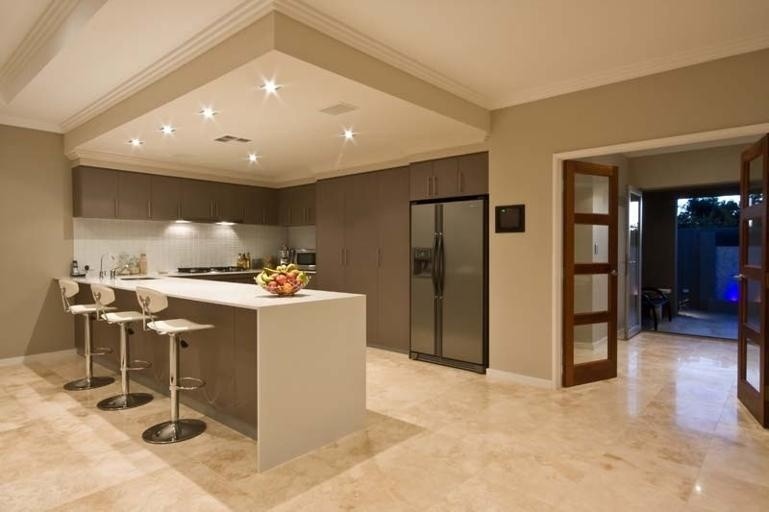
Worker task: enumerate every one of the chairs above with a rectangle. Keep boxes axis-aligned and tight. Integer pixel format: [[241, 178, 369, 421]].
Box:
[[135, 286, 207, 445], [90, 282, 157, 412], [58, 278, 116, 391]]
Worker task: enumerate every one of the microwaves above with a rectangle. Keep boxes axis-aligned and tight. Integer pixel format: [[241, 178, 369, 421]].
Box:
[[293, 249, 316, 271]]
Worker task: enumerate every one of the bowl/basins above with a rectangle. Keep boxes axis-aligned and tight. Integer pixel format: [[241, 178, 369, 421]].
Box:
[[255, 278, 311, 297]]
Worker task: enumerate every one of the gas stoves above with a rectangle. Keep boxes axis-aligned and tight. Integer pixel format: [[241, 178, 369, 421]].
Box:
[[178, 265, 241, 274]]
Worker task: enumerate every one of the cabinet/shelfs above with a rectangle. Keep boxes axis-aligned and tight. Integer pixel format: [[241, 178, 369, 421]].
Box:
[[316, 162, 410, 352], [72, 167, 116, 219], [116, 171, 179, 220], [181, 178, 208, 221], [237, 185, 280, 224], [411, 149, 489, 199], [281, 182, 316, 225], [209, 181, 235, 222]]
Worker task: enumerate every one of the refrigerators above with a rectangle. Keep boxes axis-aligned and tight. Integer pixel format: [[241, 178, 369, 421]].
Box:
[[408, 193, 489, 374]]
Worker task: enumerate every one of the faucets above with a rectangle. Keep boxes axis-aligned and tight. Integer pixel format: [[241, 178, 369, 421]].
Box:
[[98, 251, 118, 279], [110, 263, 132, 282]]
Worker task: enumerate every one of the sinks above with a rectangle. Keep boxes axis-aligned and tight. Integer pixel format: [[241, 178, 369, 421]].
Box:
[[121, 275, 162, 282]]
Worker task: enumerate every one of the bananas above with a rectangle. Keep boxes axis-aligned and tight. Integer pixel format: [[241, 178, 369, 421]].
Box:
[[257, 271, 270, 286]]
